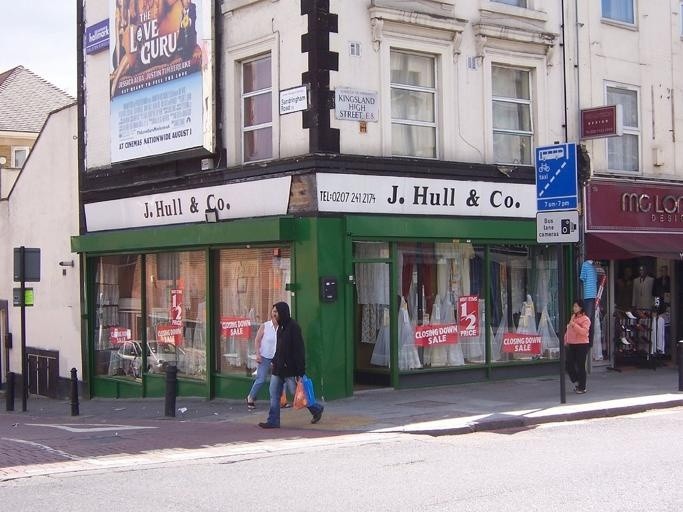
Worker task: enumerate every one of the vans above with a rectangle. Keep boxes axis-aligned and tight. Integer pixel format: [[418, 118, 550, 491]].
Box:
[[113, 337, 191, 376]]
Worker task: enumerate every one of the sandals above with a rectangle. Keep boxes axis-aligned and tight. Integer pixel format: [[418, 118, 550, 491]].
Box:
[[280, 403, 291, 408], [244, 395, 256, 409]]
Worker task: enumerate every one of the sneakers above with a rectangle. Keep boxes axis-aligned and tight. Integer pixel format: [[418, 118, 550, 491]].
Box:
[[311, 406, 324, 423], [620, 311, 653, 345], [259, 422, 280, 428], [569, 382, 586, 394]]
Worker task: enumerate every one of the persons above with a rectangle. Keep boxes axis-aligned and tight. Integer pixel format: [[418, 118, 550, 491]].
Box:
[[563, 299, 592, 394], [614, 264, 670, 306], [244, 301, 324, 429], [521, 294, 535, 333], [580, 259, 598, 347]]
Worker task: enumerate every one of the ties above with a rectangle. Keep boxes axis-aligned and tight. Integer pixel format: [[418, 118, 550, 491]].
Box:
[[641, 279, 644, 286]]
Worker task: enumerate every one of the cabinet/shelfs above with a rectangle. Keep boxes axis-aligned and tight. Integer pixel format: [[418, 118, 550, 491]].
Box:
[[607, 306, 659, 372]]
